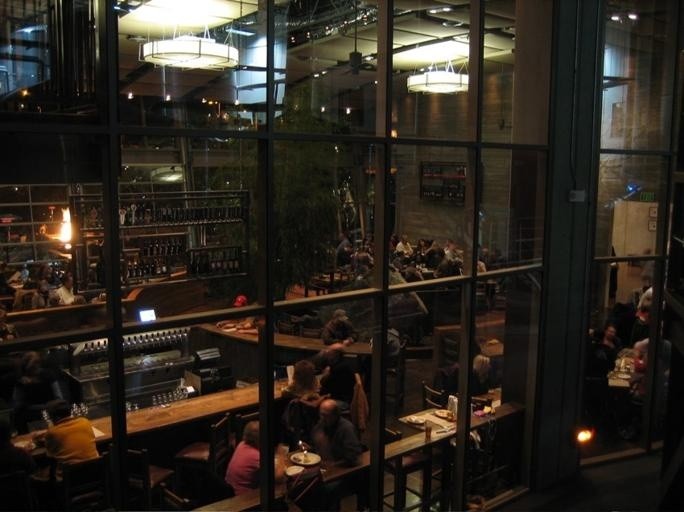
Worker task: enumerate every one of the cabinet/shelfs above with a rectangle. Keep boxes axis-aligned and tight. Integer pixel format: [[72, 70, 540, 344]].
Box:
[[187, 324, 372, 392], [74, 181, 193, 271], [418, 159, 466, 207], [0, 183, 72, 266], [8, 378, 293, 457]]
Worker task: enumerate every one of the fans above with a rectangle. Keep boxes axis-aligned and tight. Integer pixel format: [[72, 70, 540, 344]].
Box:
[[326, 0, 377, 76]]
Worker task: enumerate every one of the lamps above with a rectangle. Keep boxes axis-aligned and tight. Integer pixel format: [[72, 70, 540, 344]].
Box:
[[139, 34, 240, 72], [150, 168, 184, 185], [407, 66, 469, 95]]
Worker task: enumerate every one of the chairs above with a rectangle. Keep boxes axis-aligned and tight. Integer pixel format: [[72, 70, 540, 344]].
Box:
[[157, 481, 190, 512], [350, 242, 506, 334], [175, 412, 232, 483], [0, 457, 58, 512], [60, 452, 111, 512], [386, 339, 408, 416], [0, 272, 107, 308], [455, 392, 492, 413], [384, 427, 429, 511], [228, 412, 260, 450], [277, 320, 294, 335], [301, 326, 323, 338], [304, 266, 356, 297], [103, 439, 175, 511], [421, 380, 447, 410]]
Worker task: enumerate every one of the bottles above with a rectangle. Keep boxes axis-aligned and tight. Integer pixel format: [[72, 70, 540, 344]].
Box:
[[83, 204, 242, 278], [625, 364, 631, 373], [68, 400, 89, 419], [151, 391, 187, 407], [82, 328, 188, 352]]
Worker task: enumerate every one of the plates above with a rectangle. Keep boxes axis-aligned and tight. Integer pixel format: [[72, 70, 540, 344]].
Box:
[[287, 465, 304, 476], [407, 416, 425, 424], [435, 409, 449, 418], [290, 452, 322, 465], [14, 441, 36, 449], [618, 374, 631, 379]]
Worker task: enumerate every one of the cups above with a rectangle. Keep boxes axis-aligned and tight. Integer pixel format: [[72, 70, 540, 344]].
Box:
[[425, 421, 432, 441]]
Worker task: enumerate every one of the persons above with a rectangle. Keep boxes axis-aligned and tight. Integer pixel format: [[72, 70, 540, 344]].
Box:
[[1, 198, 673, 512]]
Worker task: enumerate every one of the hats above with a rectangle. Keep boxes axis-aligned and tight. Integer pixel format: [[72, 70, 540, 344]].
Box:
[[234, 294, 247, 307], [335, 309, 348, 322]]
